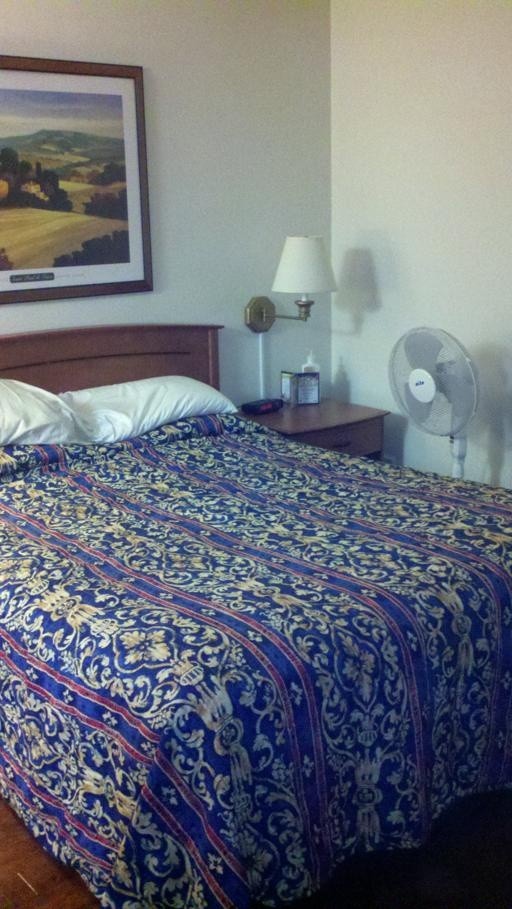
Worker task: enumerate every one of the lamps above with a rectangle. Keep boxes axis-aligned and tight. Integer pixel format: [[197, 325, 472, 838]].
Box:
[[245, 237, 338, 332]]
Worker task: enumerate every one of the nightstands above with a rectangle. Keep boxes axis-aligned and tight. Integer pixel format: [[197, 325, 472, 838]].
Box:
[[235, 399, 392, 464]]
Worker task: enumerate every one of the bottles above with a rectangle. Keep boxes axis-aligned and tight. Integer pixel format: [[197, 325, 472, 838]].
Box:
[[302, 356, 318, 373]]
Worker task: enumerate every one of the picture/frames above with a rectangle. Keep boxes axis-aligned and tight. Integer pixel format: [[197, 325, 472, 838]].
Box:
[[0, 54, 153, 304]]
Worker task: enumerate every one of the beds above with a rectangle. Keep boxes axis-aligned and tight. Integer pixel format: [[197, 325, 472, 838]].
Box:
[[1, 325, 512, 908]]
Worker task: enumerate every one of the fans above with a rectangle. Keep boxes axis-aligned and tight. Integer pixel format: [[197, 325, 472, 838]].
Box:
[[387, 326, 478, 478]]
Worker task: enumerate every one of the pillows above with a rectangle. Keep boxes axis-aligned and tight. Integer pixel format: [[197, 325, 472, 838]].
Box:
[[0, 376, 253, 478]]
